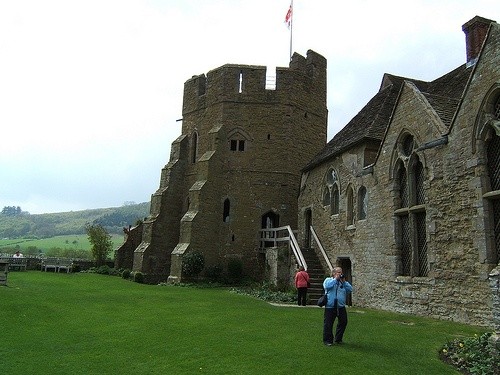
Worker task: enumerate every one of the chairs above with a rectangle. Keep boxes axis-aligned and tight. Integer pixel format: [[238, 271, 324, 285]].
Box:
[[45, 257, 70, 274]]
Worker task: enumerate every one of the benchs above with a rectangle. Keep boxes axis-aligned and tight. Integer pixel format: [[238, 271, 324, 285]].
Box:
[[8, 258, 27, 271]]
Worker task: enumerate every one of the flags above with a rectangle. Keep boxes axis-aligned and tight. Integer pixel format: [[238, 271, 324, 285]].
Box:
[[281, 1, 292, 29]]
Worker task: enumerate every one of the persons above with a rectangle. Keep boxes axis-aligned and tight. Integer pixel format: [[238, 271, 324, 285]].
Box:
[[294, 266, 309, 307], [323, 267, 352, 347], [12, 251, 23, 271]]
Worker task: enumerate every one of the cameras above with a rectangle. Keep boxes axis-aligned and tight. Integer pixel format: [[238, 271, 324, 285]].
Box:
[[339, 274, 345, 279]]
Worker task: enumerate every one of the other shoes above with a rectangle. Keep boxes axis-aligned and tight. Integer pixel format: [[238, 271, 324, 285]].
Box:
[[335, 341, 344, 345], [325, 342, 333, 346]]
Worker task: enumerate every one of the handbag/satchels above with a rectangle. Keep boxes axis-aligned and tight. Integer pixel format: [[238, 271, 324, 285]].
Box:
[[317, 294, 327, 307], [307, 282, 310, 286]]
